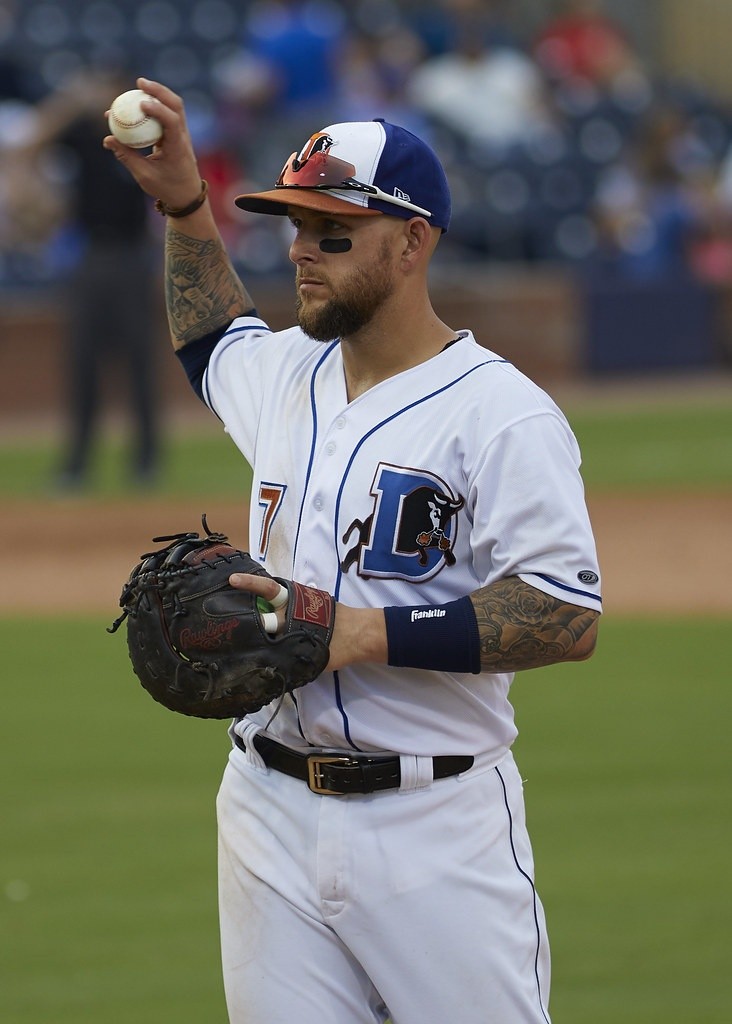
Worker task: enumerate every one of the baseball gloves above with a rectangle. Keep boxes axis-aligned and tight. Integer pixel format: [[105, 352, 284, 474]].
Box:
[[118, 531, 337, 722]]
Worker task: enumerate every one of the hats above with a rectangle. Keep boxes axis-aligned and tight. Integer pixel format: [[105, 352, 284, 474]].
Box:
[[235, 117, 452, 234]]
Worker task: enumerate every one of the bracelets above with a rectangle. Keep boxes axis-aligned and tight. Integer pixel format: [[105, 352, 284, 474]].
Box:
[[384, 595, 481, 675], [154, 181, 208, 218]]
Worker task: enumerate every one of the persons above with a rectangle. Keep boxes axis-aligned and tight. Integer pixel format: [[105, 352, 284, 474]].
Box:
[[104, 78, 604, 1024], [1, 0, 731, 487]]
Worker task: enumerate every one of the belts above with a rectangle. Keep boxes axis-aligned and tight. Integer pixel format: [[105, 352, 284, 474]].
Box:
[[234, 720, 474, 796]]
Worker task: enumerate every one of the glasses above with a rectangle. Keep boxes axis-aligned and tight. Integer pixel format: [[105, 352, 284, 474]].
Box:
[[274, 151, 432, 218]]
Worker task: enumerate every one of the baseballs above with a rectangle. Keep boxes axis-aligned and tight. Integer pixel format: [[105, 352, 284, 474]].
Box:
[[109, 87, 166, 149]]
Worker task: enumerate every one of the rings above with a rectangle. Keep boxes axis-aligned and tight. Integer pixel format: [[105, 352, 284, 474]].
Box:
[[262, 613, 278, 633], [267, 584, 289, 607]]
